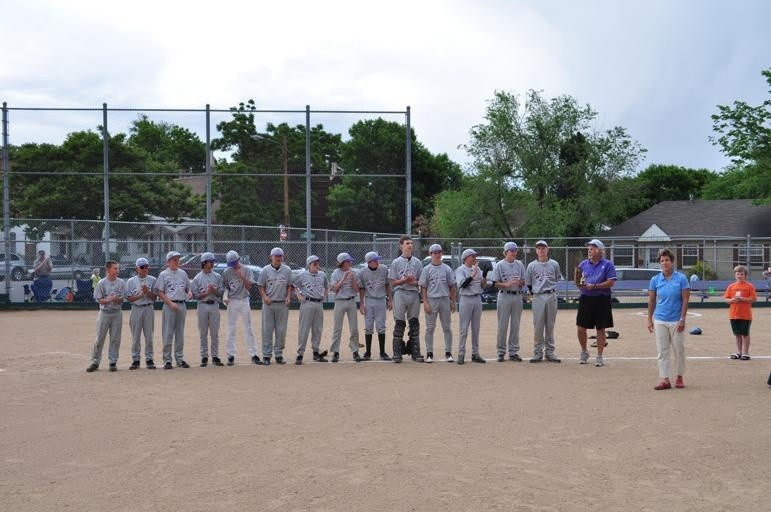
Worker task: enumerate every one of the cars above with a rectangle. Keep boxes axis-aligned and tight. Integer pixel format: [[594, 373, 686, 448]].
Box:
[[99, 251, 262, 282], [280, 262, 305, 280], [27, 258, 94, 280]]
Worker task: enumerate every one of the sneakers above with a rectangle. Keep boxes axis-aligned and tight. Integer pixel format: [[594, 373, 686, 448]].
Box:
[[731, 354, 749, 360], [146, 360, 156, 370], [411, 352, 560, 363], [295, 355, 302, 364], [130, 360, 139, 368], [212, 356, 224, 366], [654, 383, 670, 389], [228, 356, 234, 365], [87, 364, 98, 372], [596, 356, 604, 367], [109, 363, 116, 370], [675, 378, 684, 388], [164, 363, 172, 369], [252, 355, 286, 365], [312, 351, 400, 363], [179, 361, 190, 368], [579, 351, 589, 364], [200, 357, 207, 366]]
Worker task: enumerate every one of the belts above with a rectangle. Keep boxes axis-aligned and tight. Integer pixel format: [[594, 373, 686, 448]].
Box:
[[545, 290, 554, 293], [307, 297, 321, 302], [206, 301, 215, 304], [143, 303, 150, 306], [173, 300, 185, 303], [501, 290, 522, 294], [345, 296, 354, 300]]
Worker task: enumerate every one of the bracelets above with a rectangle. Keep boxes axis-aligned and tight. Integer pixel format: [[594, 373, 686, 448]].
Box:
[[594, 284, 597, 289], [680, 319, 685, 322]]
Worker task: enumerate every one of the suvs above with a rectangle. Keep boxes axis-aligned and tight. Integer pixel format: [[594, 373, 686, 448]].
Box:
[[615, 268, 664, 292], [1, 251, 30, 282], [421, 254, 499, 288]]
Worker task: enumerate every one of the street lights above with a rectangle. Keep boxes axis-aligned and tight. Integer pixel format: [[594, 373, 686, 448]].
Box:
[[248, 129, 291, 262]]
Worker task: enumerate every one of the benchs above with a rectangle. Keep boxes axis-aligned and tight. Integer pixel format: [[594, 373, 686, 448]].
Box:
[[689, 278, 771, 304], [523, 279, 650, 308]]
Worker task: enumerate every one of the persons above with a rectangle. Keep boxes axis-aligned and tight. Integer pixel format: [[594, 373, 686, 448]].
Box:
[[33, 250, 53, 278], [722, 264, 757, 360], [90, 267, 102, 296], [690, 271, 699, 281], [574, 239, 618, 367], [154, 250, 194, 369], [647, 249, 691, 390], [191, 234, 525, 367], [524, 240, 562, 363], [86, 260, 127, 372], [125, 258, 158, 370]]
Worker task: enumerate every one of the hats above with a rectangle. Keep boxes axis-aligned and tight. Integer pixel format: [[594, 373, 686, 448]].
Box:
[[167, 251, 180, 260], [271, 247, 284, 257], [366, 252, 381, 263], [337, 253, 353, 262], [430, 244, 441, 253], [536, 240, 547, 247], [226, 251, 240, 267], [504, 242, 519, 250], [307, 255, 318, 264], [461, 249, 479, 259], [201, 252, 215, 262], [136, 258, 148, 266], [586, 239, 602, 249]]
